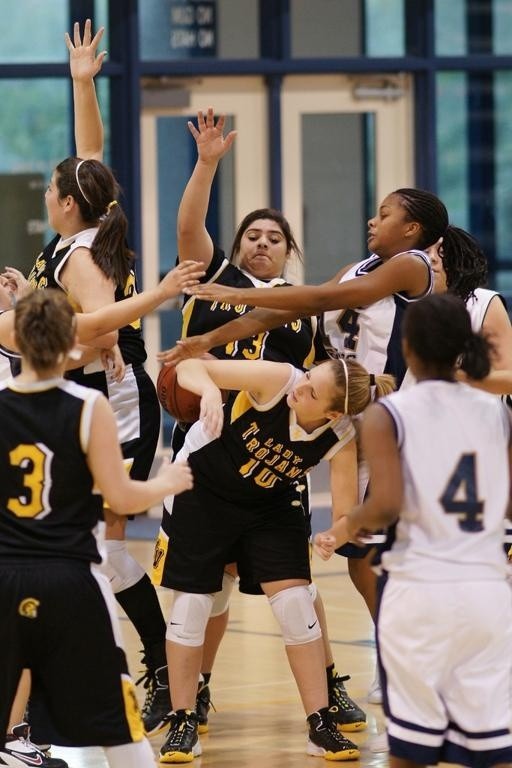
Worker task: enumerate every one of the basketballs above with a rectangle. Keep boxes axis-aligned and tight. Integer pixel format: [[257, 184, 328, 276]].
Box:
[[157, 352, 230, 424]]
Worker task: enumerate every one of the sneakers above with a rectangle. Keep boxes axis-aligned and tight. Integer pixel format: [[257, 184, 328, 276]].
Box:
[[140, 662, 210, 762], [306, 663, 382, 760], [0, 723, 68, 768]]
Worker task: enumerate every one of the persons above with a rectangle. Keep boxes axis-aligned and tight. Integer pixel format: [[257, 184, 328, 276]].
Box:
[[1, 258, 208, 768], [176, 107, 366, 736], [427, 227, 511, 398], [159, 352, 393, 761], [0, 289, 193, 766], [159, 190, 448, 701], [340, 295, 512, 767], [26, 18, 205, 735]]
